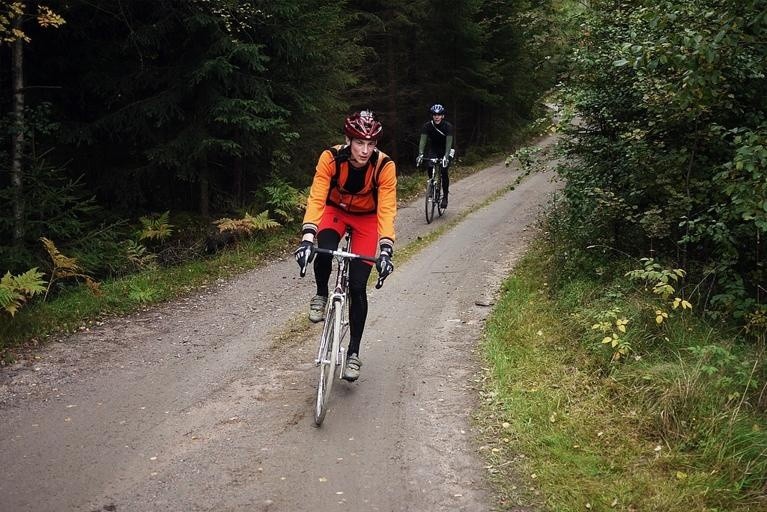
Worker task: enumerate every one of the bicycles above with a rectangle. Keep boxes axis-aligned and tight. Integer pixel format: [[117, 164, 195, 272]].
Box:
[[289, 243, 385, 427], [415, 154, 451, 225]]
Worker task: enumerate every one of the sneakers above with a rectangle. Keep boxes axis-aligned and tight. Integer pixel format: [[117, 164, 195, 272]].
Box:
[[344, 353, 360, 381], [441, 198, 448, 208], [309, 294, 327, 323]]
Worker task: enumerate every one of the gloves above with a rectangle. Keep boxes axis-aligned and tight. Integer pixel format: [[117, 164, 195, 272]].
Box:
[[416, 156, 423, 164], [294, 241, 315, 268], [377, 251, 394, 278]]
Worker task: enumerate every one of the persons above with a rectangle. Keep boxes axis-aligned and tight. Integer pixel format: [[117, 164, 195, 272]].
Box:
[[416, 104, 456, 208], [295, 110, 397, 382]]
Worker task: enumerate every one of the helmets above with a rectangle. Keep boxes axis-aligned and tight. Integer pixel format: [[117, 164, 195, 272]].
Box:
[[431, 104, 446, 115], [344, 110, 383, 140]]
